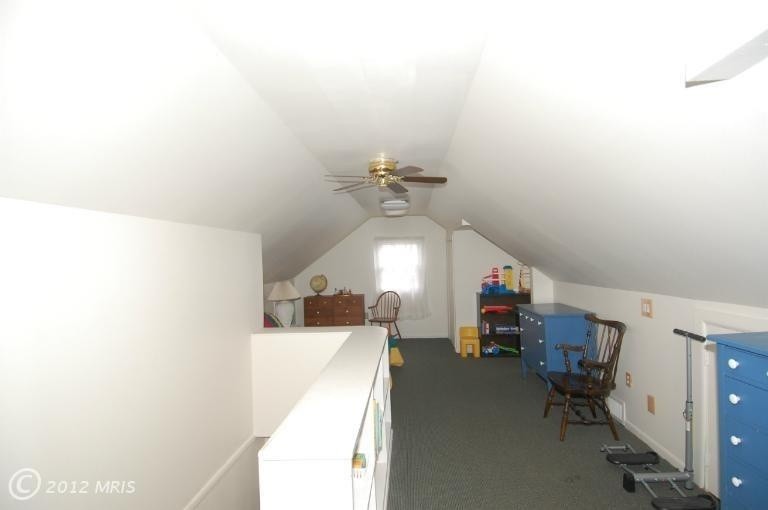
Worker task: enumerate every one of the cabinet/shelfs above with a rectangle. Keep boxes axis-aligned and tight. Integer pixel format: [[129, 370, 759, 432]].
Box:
[[706, 331, 768, 510], [477, 292, 532, 358], [515, 302, 596, 399], [250, 325, 394, 510], [303, 294, 365, 327]]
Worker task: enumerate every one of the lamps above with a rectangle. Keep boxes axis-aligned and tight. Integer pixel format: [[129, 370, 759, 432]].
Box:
[[267, 280, 302, 327], [379, 198, 411, 217]]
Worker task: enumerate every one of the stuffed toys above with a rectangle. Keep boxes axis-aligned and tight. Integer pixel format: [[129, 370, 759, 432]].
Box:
[[481, 265, 516, 295], [481, 304, 513, 314], [482, 338, 520, 356]]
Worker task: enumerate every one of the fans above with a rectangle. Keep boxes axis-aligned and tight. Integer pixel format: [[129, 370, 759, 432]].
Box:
[[323, 157, 447, 193]]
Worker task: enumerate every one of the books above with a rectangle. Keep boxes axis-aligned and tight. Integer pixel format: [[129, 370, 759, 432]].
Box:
[[373, 399, 384, 455], [480, 318, 519, 336]]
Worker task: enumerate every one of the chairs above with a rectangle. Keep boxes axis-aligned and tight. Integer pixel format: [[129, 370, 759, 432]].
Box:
[[368, 291, 402, 342], [543, 313, 627, 441], [459, 326, 480, 358]]
